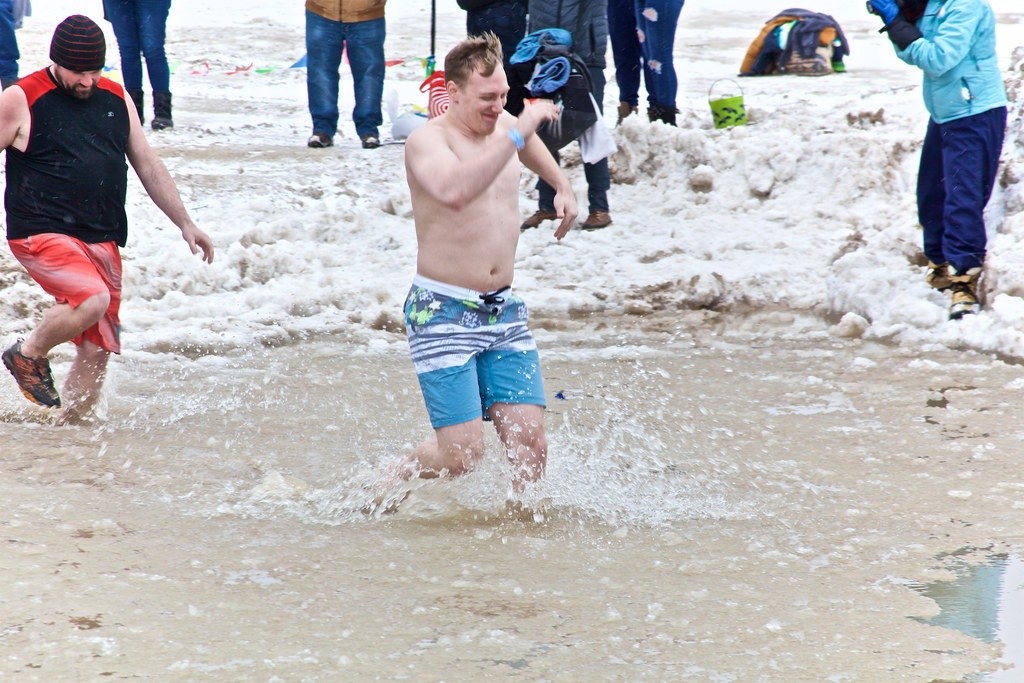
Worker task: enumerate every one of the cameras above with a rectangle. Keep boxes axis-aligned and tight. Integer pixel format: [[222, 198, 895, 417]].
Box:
[[866, 0, 903, 14]]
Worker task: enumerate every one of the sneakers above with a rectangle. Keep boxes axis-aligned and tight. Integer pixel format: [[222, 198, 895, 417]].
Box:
[[943, 264, 984, 317], [581, 212, 612, 231], [924, 259, 954, 289], [2, 338, 61, 409], [519, 210, 562, 230]]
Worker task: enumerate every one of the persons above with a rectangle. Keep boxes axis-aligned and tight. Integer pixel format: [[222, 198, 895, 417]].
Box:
[[608, 0, 685, 127], [391, 29, 578, 492], [0, 14, 214, 410], [0, 0, 31, 91], [455, 0, 527, 117], [304, 0, 387, 148], [868, 0, 1007, 318], [521, 0, 612, 232], [100, 0, 173, 129]]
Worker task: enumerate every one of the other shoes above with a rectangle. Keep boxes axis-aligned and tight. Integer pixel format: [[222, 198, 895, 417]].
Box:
[[647, 109, 678, 128], [362, 134, 379, 147], [615, 100, 638, 125], [309, 131, 333, 147]]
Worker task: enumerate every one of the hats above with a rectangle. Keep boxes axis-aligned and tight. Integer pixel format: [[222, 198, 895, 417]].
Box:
[[50, 15, 106, 72]]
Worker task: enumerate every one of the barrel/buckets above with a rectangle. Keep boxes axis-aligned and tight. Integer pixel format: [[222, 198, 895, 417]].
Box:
[[707, 78, 748, 131]]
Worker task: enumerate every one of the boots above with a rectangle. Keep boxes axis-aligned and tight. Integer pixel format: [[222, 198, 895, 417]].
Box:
[[151, 89, 174, 130], [128, 87, 144, 128]]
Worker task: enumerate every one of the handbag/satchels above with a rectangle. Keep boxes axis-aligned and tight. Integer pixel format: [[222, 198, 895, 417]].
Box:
[[528, 72, 597, 153]]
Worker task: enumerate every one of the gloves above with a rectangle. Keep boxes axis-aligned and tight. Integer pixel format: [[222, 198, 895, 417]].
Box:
[[871, 0, 900, 26]]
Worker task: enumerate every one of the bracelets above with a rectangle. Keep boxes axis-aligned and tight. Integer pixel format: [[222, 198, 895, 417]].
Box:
[[505, 129, 524, 148]]
